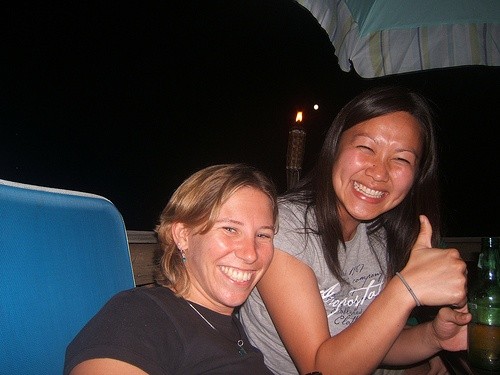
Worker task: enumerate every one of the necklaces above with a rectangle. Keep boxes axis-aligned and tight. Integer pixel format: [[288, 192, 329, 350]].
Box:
[[177, 290, 248, 358]]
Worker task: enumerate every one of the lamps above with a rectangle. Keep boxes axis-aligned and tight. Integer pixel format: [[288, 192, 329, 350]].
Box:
[[286, 110, 307, 190]]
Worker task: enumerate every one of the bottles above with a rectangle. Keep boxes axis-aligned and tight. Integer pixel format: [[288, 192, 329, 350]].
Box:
[[467, 237, 500, 375]]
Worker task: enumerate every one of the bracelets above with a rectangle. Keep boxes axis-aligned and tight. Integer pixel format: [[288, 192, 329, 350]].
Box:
[[396, 272, 421, 307]]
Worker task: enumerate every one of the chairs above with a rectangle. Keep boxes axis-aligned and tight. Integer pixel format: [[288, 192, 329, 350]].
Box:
[[0, 178, 136, 374]]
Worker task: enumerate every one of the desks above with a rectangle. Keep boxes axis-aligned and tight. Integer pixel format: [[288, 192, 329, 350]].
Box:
[[416, 262, 500, 375]]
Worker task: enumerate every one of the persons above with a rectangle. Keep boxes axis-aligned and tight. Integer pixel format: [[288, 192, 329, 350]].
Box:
[[64, 165, 280, 375], [238, 85, 500, 375]]
